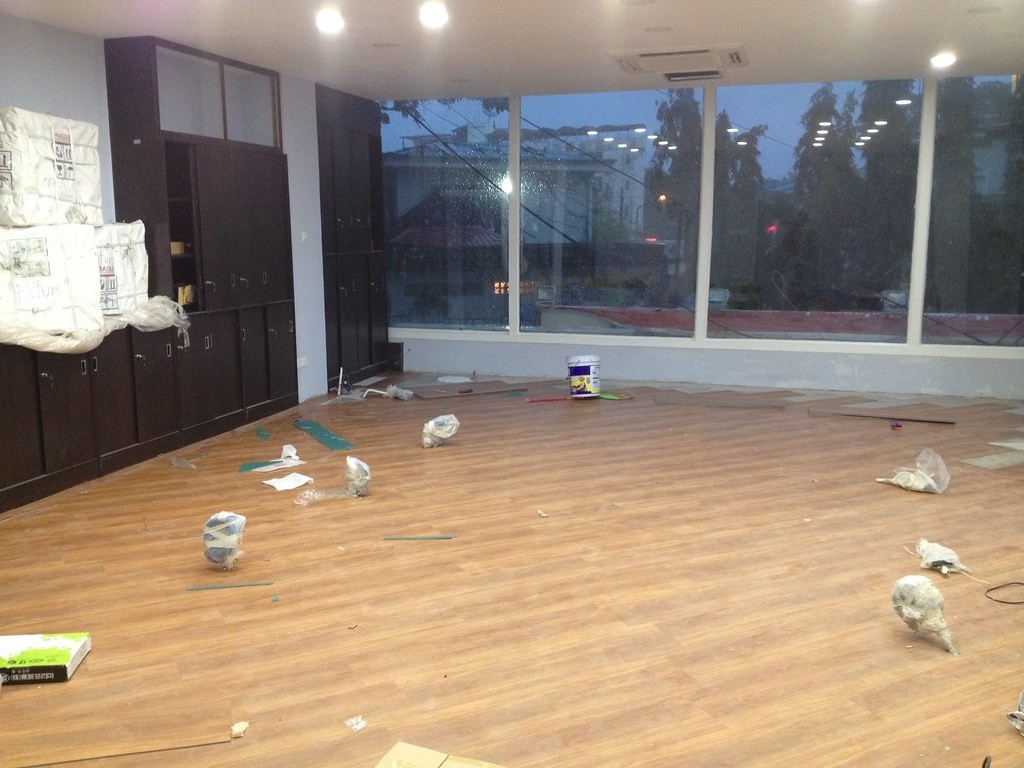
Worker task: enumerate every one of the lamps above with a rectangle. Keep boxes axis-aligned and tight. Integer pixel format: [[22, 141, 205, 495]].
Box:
[[881, 290, 908, 311], [708, 288, 731, 311], [536, 286, 555, 306]]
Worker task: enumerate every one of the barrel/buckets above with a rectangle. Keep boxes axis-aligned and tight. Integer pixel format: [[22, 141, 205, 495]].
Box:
[[566, 355, 601, 397]]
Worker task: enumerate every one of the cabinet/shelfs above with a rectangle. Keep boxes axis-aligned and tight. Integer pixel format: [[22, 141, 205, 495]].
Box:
[[314, 83, 396, 392], [0, 35, 300, 514]]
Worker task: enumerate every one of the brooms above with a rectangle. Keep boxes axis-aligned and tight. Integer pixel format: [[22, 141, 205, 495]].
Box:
[[526, 390, 635, 403]]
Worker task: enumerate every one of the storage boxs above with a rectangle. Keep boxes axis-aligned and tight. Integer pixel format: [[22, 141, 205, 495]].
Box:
[[0, 632, 92, 685]]
